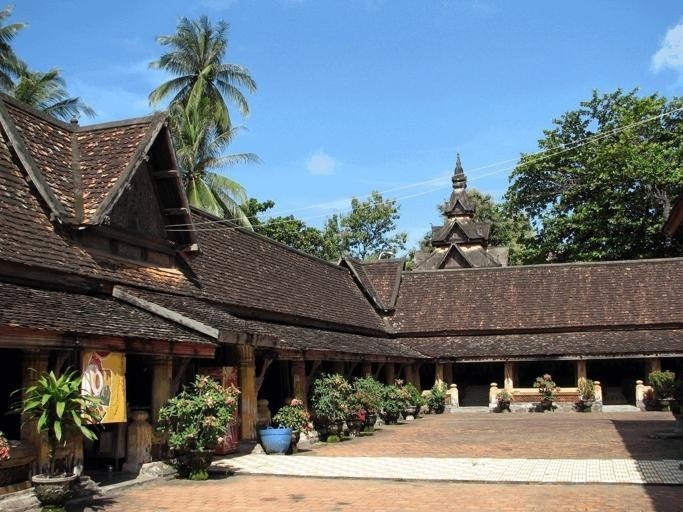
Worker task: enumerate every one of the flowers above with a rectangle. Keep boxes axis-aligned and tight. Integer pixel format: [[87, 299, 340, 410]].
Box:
[[533, 374, 560, 401], [157, 376, 240, 447], [271, 371, 414, 423]]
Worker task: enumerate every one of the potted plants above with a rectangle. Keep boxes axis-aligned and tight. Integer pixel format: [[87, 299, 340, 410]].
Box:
[[11, 367, 102, 502], [578, 378, 595, 408], [496, 390, 511, 412], [648, 369, 677, 408], [428, 380, 446, 416]]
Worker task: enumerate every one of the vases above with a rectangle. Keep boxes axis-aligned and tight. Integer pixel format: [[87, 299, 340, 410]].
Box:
[[178, 446, 214, 481], [282, 407, 420, 444], [541, 401, 552, 412]]
